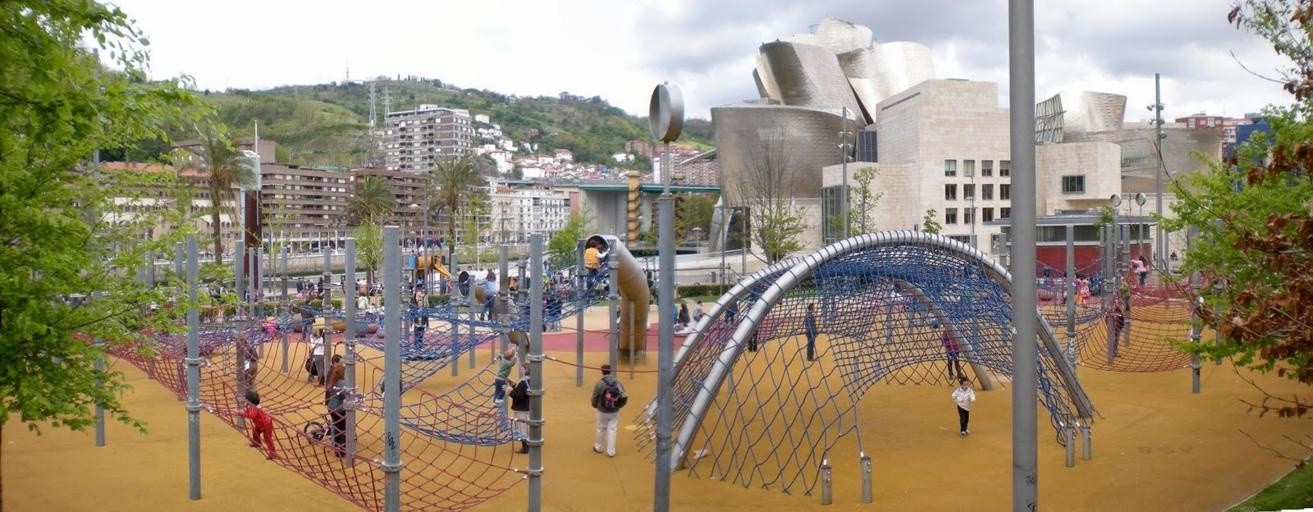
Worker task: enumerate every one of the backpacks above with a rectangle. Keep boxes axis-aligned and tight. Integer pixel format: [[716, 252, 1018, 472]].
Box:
[[602, 376, 625, 411]]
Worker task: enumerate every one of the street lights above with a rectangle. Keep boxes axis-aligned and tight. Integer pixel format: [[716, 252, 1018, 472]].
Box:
[[710, 202, 726, 303], [836, 105, 856, 241], [1110, 194, 1121, 274], [1134, 193, 1144, 262], [1146, 72, 1167, 294], [645, 81, 685, 512], [407, 201, 428, 296], [691, 225, 702, 265]]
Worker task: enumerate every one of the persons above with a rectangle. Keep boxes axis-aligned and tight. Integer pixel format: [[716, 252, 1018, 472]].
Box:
[[942, 330, 965, 380], [951, 377, 975, 436], [323, 354, 346, 458], [357, 278, 382, 295], [297, 277, 324, 299], [310, 329, 324, 387], [677, 297, 758, 352], [590, 364, 628, 456], [409, 276, 430, 327], [492, 350, 518, 407], [508, 276, 519, 297], [301, 300, 315, 341], [242, 340, 259, 392], [440, 276, 452, 293], [584, 239, 611, 300], [501, 364, 530, 454], [486, 268, 497, 280], [210, 284, 228, 304], [804, 303, 818, 362], [478, 277, 497, 321], [358, 293, 384, 320], [1043, 255, 1148, 358], [410, 250, 417, 257], [230, 391, 277, 460], [542, 288, 562, 332], [199, 348, 211, 367]]
